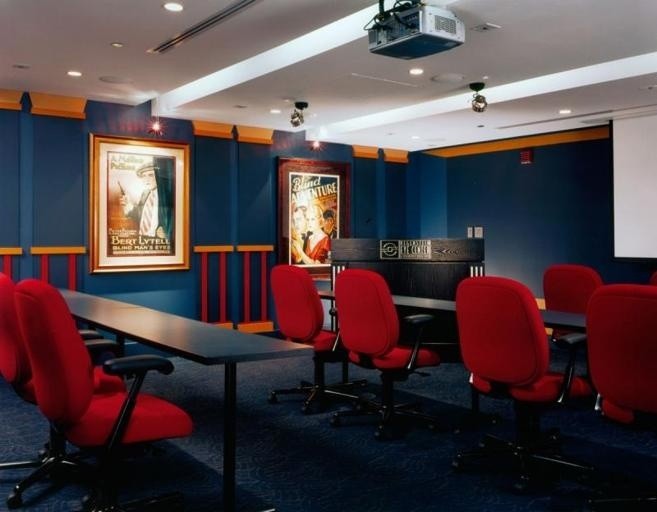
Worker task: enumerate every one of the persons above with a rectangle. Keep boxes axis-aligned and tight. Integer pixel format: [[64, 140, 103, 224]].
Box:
[[119, 160, 174, 250], [291, 205, 337, 265]]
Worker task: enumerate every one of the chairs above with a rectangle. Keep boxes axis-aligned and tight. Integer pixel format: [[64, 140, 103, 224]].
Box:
[[0, 245, 655, 512]]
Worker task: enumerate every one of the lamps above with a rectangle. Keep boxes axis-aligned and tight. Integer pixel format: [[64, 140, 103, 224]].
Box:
[[290, 99, 308, 128], [466, 81, 488, 115]]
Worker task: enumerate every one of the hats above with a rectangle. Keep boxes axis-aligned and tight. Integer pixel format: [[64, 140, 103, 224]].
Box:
[[137, 162, 161, 176]]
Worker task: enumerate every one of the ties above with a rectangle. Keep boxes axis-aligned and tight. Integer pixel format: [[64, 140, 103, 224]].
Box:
[[139, 190, 157, 235]]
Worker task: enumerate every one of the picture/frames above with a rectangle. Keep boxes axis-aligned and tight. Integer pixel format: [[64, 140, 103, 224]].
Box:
[[272, 153, 352, 280], [86, 130, 192, 276]]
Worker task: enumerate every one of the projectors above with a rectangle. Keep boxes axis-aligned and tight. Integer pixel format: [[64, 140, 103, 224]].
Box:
[[368, 6, 465, 60]]
